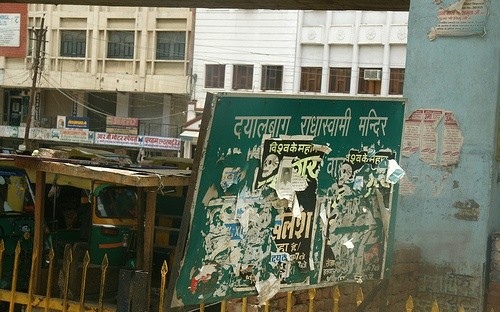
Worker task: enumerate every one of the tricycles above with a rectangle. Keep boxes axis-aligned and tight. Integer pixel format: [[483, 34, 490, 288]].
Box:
[[25, 168, 146, 270], [0, 164, 59, 312]]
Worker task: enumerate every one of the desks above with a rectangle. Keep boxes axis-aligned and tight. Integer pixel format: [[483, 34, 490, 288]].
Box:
[[12, 155, 194, 295]]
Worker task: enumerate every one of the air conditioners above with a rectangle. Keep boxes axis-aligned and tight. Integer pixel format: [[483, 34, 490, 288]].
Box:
[[364, 69, 381, 81]]
